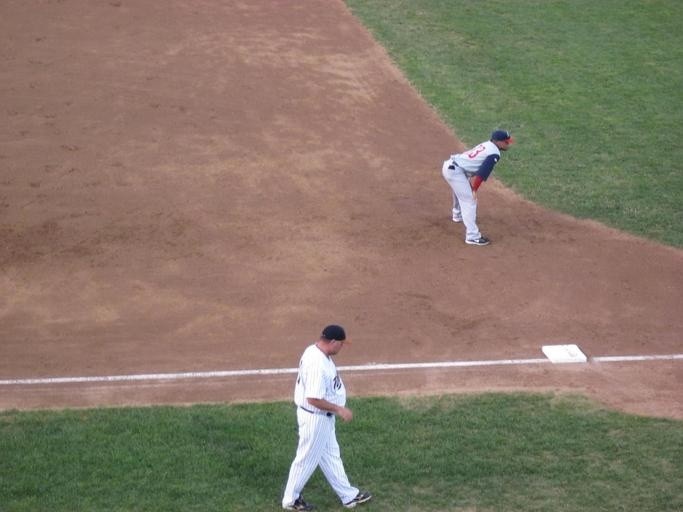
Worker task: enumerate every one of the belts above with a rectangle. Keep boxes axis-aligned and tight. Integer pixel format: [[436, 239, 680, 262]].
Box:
[[302, 406, 333, 416], [453, 162, 458, 167]]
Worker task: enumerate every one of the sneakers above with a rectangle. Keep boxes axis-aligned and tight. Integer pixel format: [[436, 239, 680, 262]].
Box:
[[465, 237, 490, 245], [343, 491, 371, 508], [282, 497, 314, 512], [453, 216, 462, 222]]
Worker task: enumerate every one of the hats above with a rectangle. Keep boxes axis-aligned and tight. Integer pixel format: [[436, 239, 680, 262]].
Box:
[[322, 325, 353, 345], [492, 131, 515, 144]]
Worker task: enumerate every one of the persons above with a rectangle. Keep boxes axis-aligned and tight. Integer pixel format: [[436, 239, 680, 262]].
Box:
[[441, 130, 517, 246], [280, 323, 372, 511]]
